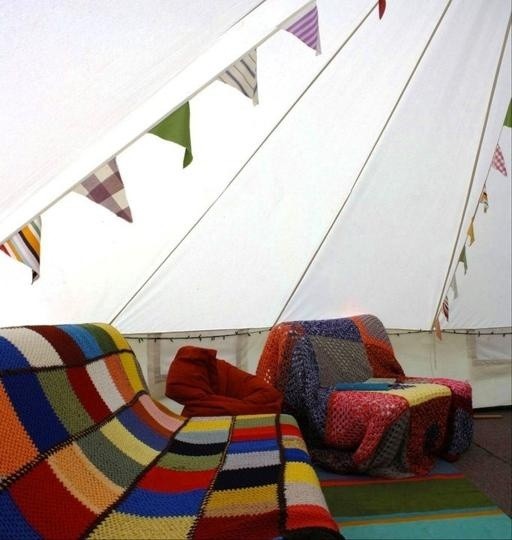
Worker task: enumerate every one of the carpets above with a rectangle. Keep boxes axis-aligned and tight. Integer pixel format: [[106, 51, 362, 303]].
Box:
[[312, 458, 512, 540]]
